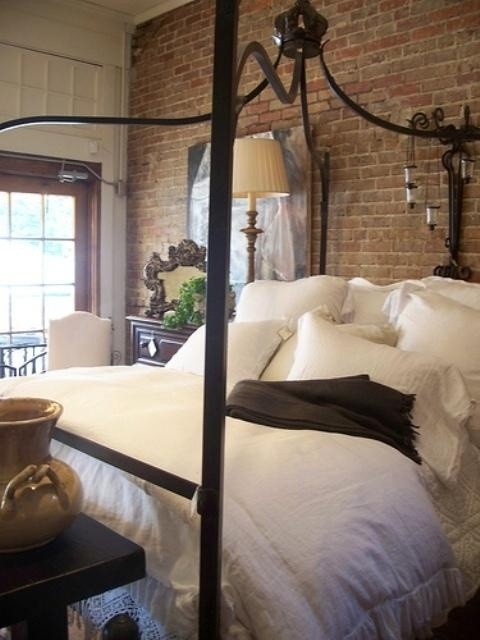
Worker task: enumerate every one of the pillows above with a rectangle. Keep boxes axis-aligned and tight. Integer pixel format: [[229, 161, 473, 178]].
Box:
[[158, 273, 480, 434]]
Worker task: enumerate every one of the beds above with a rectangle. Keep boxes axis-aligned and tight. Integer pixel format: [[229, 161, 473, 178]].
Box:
[[0, 283, 480, 637]]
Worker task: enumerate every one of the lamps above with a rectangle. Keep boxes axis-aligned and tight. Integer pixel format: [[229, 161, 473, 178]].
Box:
[[230, 138, 290, 289]]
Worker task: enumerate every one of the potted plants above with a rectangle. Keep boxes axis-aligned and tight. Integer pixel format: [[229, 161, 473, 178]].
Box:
[[163, 276, 236, 330]]
